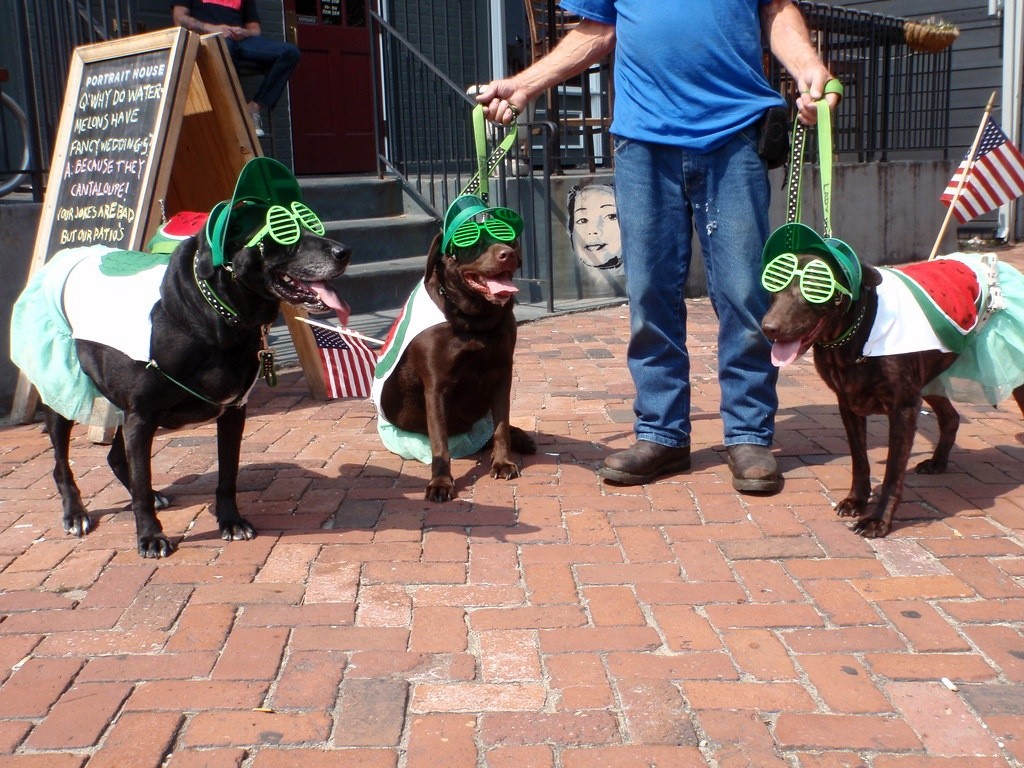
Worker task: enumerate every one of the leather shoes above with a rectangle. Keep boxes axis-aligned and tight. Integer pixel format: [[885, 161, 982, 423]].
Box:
[[727, 443, 779, 491], [602, 440, 691, 485]]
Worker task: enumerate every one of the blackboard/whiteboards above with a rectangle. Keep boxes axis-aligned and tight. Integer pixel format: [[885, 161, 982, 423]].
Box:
[[28, 26, 202, 287]]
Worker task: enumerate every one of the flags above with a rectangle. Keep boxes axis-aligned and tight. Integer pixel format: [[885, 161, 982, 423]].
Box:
[[306, 318, 379, 399], [939, 113, 1024, 224]]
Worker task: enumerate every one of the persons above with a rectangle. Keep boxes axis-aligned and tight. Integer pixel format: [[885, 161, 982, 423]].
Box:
[[476, 0, 845, 492], [173, 0, 303, 136]]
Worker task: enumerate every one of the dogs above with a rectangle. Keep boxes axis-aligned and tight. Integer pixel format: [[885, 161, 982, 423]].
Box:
[[761, 244, 1024, 540], [370, 217, 536, 503], [9, 201, 351, 560]]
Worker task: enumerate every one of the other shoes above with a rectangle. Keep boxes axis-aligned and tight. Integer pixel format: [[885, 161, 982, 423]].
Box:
[[251, 112, 265, 137]]
